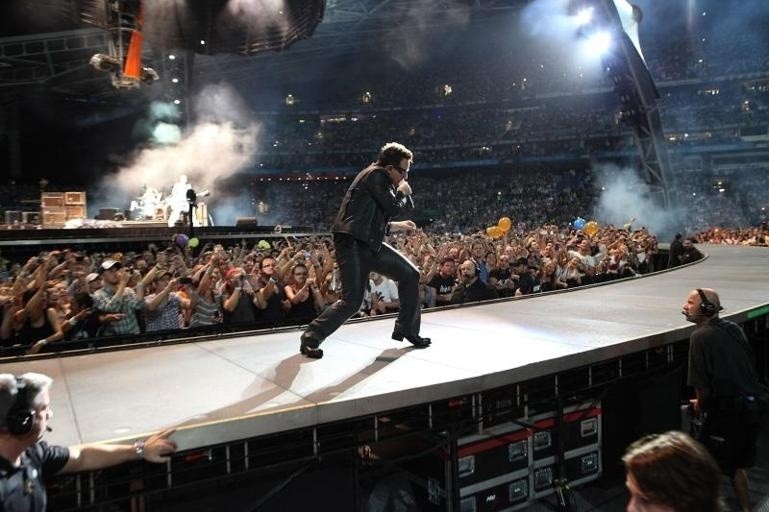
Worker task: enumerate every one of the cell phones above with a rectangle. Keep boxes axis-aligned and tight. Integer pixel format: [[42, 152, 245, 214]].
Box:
[[127, 259, 133, 274]]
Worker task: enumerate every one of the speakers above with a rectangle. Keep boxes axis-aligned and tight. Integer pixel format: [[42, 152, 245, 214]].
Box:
[[22, 212, 42, 225], [236, 216, 257, 226], [2, 211, 22, 224], [100, 207, 119, 220]]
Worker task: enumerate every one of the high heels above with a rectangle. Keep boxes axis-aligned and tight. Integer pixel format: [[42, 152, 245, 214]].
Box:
[[392, 330, 431, 347], [300, 338, 323, 359]]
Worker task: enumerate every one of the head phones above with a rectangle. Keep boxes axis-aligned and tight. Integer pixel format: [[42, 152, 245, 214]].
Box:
[[6, 375, 36, 437], [459, 257, 480, 276], [696, 288, 723, 317]]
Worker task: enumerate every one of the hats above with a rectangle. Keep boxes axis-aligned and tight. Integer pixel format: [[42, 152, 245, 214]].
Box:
[[508, 257, 527, 266], [97, 259, 122, 274], [85, 273, 100, 284], [152, 269, 172, 281]]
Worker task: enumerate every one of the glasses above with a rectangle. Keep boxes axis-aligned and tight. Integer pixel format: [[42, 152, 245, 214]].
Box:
[[393, 165, 406, 176], [262, 263, 275, 269]]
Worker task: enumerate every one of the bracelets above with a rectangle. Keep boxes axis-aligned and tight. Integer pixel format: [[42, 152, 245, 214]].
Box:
[[133, 438, 146, 461]]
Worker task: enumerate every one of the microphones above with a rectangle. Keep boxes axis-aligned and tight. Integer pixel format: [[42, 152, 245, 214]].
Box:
[[681, 311, 705, 317], [46, 426, 52, 431], [398, 180, 415, 209]]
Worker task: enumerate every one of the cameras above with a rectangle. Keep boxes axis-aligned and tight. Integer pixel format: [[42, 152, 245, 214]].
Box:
[[239, 270, 253, 281], [180, 278, 192, 284]]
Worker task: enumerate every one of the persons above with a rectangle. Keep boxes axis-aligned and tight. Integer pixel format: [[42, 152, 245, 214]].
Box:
[[167, 174, 192, 224], [1, 371, 181, 512], [621, 430, 720, 511], [682, 286, 759, 511], [299, 141, 432, 358], [223, 16, 768, 245], [0, 216, 695, 356]]
